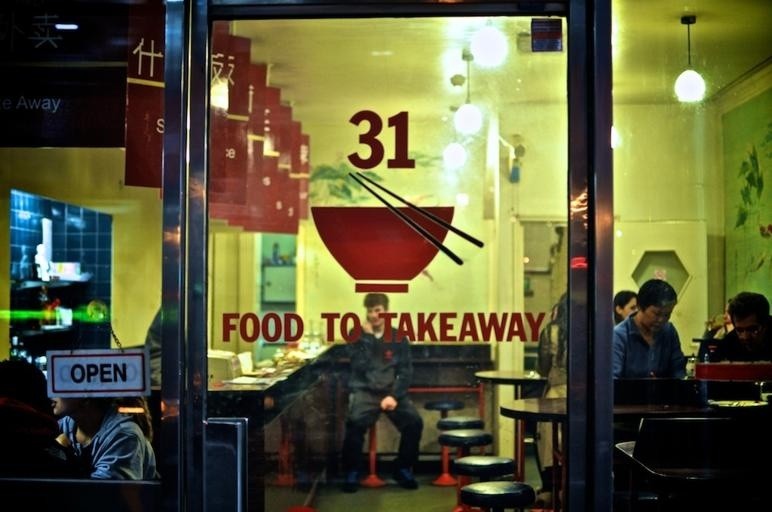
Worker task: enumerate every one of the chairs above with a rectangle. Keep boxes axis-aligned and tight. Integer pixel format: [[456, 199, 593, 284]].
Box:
[[616, 417, 739, 512]]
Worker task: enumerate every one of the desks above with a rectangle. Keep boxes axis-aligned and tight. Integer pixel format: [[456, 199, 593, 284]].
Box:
[[475, 368, 549, 479], [501, 397, 771, 512]]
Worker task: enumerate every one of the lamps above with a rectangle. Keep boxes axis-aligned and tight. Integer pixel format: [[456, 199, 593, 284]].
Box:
[[452, 47, 485, 135], [468, 17, 509, 68], [673, 14, 707, 101], [443, 99, 470, 172]]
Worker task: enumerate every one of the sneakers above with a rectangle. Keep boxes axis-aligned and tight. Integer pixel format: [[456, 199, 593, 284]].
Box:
[[394, 467, 419, 489], [341, 470, 361, 493]]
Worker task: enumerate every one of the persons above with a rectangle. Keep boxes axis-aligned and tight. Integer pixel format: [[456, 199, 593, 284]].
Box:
[[0, 355, 83, 479], [698, 298, 735, 341], [144, 304, 164, 386], [707, 291, 772, 394], [534, 291, 569, 466], [613, 289, 641, 324], [341, 293, 423, 466], [49, 394, 163, 482], [613, 278, 689, 382]]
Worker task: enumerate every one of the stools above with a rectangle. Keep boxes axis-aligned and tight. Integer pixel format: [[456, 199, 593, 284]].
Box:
[[360, 424, 387, 488], [439, 429, 492, 511], [425, 400, 466, 486], [453, 455, 515, 511], [447, 478, 561, 507], [460, 481, 535, 512], [437, 416, 492, 485]]
